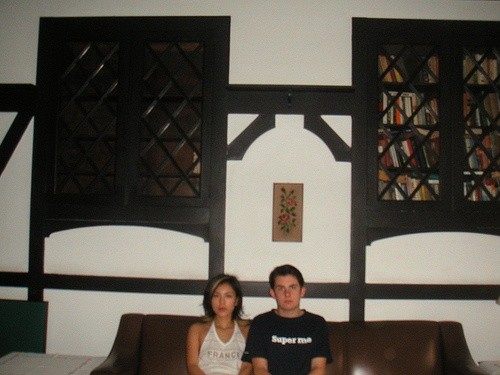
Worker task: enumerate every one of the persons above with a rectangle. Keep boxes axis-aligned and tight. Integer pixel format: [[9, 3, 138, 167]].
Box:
[[185, 273, 254, 375], [241, 264, 333, 375]]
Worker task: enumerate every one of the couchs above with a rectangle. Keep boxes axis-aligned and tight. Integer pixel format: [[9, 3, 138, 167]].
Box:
[[90, 313, 489, 375]]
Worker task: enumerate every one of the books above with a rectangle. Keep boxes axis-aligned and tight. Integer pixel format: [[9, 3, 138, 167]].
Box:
[[378, 54, 438, 84], [464, 93, 499, 124], [466, 174, 500, 199], [377, 92, 439, 124], [378, 174, 440, 201], [464, 131, 500, 167], [462, 56, 498, 84], [378, 129, 441, 170]]
